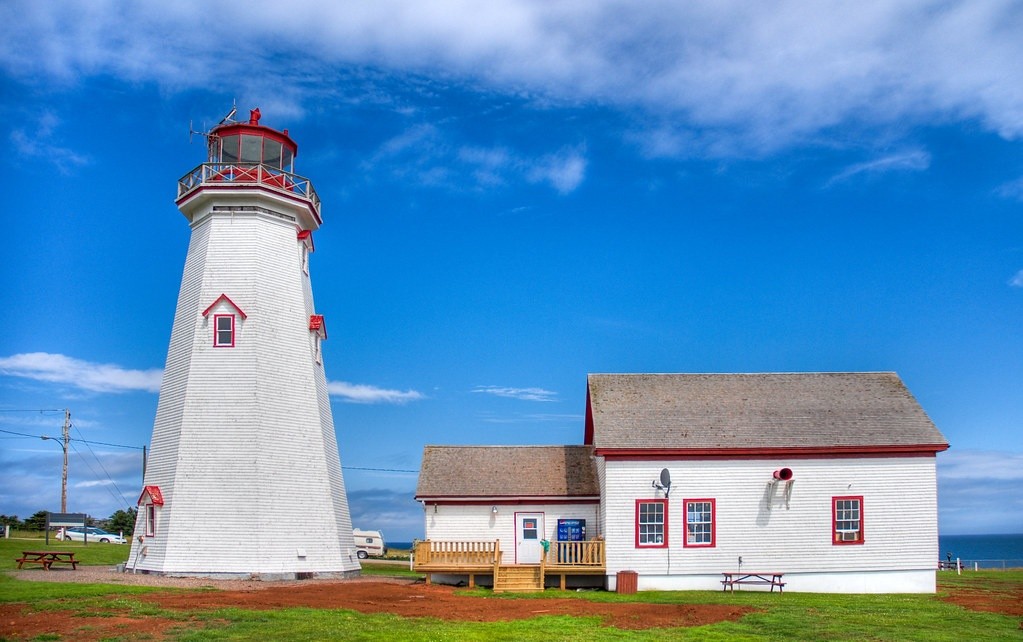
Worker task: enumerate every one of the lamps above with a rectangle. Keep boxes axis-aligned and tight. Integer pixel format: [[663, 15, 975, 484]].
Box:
[[492, 506, 498, 513]]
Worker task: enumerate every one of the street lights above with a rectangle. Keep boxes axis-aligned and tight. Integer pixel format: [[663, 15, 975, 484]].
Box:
[[41, 436, 68, 541]]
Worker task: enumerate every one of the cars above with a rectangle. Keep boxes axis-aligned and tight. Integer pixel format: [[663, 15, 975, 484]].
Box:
[[0, 526, 5, 537], [55, 526, 127, 544]]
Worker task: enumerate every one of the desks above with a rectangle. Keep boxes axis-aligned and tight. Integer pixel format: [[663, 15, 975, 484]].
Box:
[[938, 561, 964, 571], [16, 550, 77, 572], [722, 572, 784, 596]]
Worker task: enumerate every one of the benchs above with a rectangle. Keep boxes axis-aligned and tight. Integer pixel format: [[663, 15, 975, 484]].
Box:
[[939, 565, 964, 569], [54, 559, 82, 564], [719, 580, 787, 587], [16, 558, 53, 564]]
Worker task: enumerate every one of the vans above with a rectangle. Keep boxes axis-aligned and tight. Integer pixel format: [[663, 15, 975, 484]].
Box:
[[351, 528, 388, 560]]
